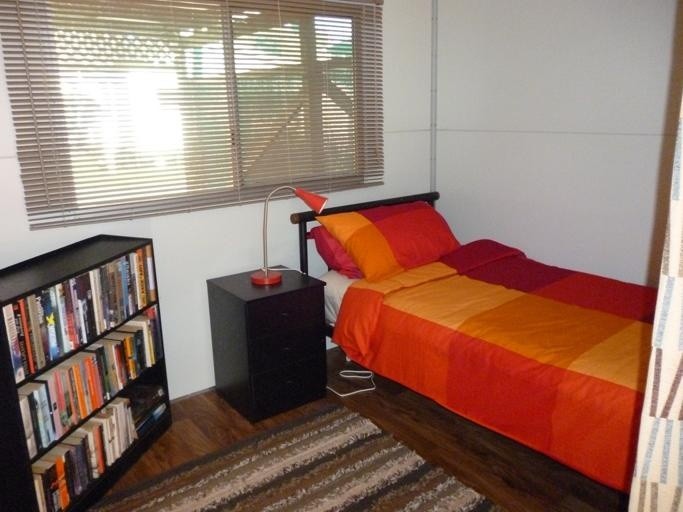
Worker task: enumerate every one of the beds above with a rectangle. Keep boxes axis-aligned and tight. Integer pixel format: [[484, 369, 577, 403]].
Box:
[[290, 191, 659, 494]]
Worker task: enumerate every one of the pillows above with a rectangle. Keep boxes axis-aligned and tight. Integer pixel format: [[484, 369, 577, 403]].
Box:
[[310, 225, 366, 281], [316, 201, 461, 283]]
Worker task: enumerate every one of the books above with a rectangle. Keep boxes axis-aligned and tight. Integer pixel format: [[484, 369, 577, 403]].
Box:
[[0, 243, 168, 512]]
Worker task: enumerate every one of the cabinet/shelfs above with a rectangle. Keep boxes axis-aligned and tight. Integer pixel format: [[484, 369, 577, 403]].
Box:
[[0, 234, 173, 511]]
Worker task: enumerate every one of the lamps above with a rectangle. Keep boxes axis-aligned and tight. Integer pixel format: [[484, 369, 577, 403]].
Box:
[[250, 186, 328, 284]]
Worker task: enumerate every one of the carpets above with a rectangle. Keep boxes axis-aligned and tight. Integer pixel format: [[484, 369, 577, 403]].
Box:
[[90, 406, 510, 512]]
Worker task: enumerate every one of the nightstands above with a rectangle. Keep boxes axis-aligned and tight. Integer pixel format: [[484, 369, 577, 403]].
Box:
[[206, 263, 325, 426]]
